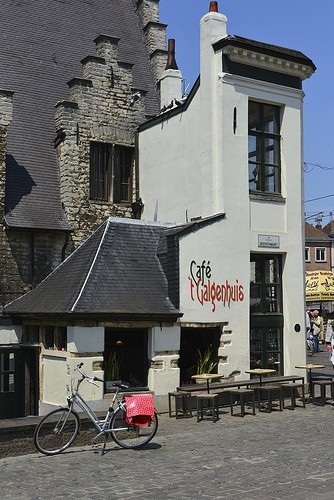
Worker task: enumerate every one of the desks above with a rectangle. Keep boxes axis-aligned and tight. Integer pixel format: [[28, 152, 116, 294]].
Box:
[[244, 370, 277, 408], [191, 374, 224, 416], [293, 364, 325, 403]]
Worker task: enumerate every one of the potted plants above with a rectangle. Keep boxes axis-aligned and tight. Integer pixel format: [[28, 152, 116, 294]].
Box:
[[194, 345, 221, 384]]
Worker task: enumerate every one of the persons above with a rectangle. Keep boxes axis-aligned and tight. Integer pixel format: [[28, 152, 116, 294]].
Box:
[[305, 309, 322, 353], [327, 321, 334, 371]]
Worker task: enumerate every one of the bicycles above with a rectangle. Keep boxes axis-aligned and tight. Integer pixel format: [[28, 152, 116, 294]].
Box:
[[33, 362, 160, 455], [306, 337, 315, 357]]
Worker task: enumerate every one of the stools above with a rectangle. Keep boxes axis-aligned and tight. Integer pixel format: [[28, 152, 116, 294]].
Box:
[[196, 394, 219, 423], [308, 374, 334, 407], [250, 386, 283, 413], [228, 388, 256, 417], [280, 383, 305, 410], [168, 392, 191, 419]]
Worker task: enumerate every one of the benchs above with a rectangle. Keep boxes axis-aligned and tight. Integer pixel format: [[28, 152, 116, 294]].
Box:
[[177, 375, 305, 412]]
